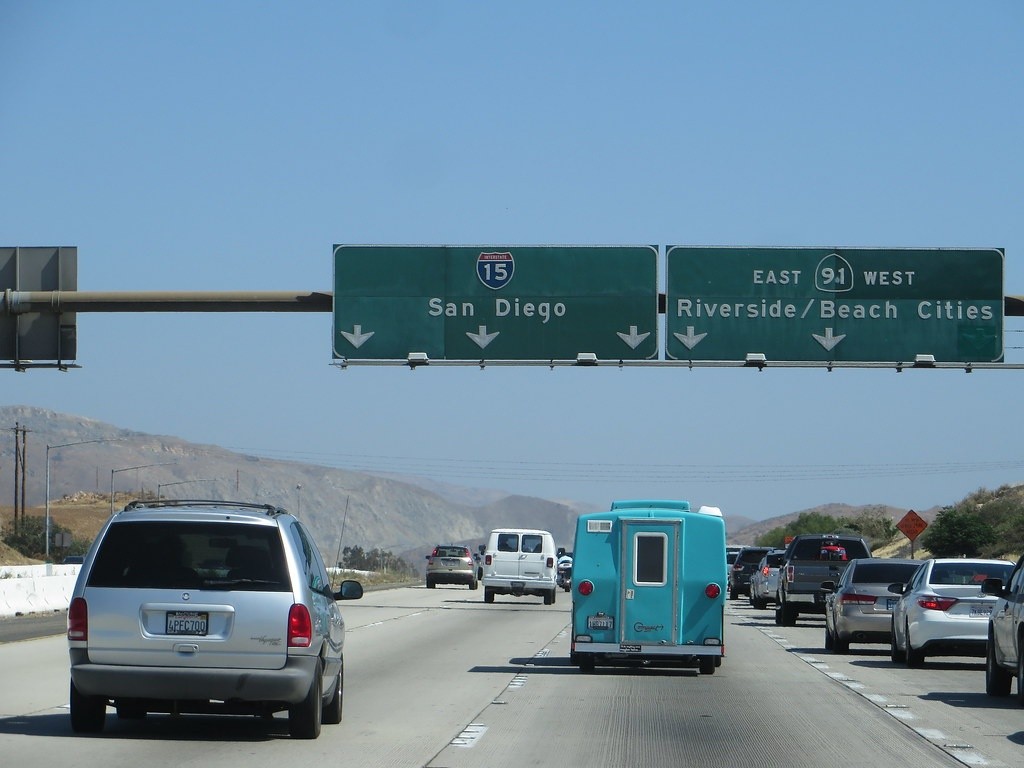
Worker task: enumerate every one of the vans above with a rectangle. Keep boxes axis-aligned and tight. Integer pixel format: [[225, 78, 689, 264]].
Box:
[[480, 529, 555, 605], [570, 500, 725, 675]]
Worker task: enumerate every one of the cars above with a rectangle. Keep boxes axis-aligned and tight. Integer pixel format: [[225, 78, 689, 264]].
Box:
[[981, 554, 1024, 706], [888, 559, 1017, 668], [820, 558, 925, 654], [750, 550, 787, 609]]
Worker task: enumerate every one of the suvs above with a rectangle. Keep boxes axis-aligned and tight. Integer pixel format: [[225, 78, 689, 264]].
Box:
[[68, 500, 363, 738], [730, 547, 777, 600], [776, 534, 874, 626], [426, 545, 478, 589]]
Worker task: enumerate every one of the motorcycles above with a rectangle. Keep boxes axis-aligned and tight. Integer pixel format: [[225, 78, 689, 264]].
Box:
[[558, 556, 572, 592]]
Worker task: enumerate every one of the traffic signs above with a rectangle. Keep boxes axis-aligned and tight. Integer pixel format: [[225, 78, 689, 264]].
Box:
[[332, 244, 658, 366], [665, 244, 1004, 369]]
